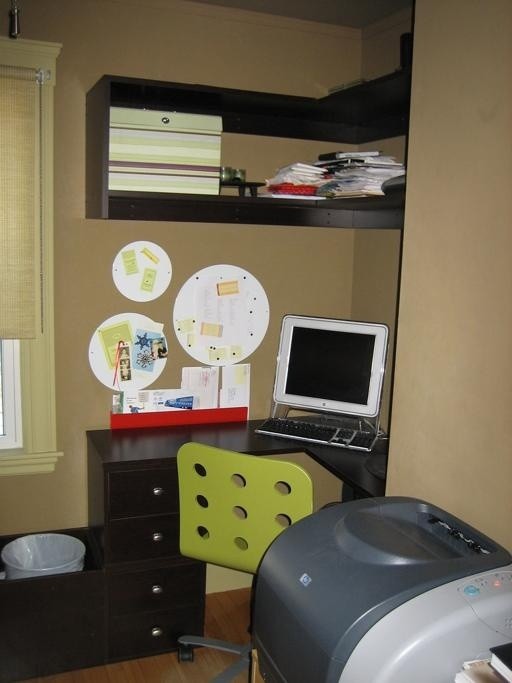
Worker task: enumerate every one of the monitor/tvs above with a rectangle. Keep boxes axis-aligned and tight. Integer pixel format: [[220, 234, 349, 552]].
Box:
[[273, 314, 389, 431]]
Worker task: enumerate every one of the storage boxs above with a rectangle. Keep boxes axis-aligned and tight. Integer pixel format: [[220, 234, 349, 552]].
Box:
[[0, 527, 108, 683], [108, 105, 223, 196]]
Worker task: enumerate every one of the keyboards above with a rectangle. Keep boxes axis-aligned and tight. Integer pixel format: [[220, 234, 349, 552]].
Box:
[[255, 417, 377, 452]]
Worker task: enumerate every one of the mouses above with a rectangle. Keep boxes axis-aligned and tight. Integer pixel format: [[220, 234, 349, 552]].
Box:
[[367, 458, 386, 480]]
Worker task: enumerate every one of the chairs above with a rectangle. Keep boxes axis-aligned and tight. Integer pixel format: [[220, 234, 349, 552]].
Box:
[[176, 441, 314, 683]]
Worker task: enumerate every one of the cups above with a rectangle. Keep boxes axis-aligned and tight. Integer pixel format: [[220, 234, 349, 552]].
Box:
[[233, 168, 246, 182], [220, 164, 232, 181]]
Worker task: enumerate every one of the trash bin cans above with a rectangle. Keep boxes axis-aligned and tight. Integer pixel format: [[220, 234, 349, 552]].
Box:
[[1, 533, 86, 581]]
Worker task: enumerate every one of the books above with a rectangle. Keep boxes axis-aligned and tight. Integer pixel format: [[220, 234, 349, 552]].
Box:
[[452, 642, 512, 682], [262, 142, 406, 200]]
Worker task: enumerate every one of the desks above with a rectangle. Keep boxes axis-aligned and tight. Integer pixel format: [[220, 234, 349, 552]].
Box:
[[85, 415, 389, 665]]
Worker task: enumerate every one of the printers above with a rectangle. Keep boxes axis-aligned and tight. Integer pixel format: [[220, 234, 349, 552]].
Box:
[[251, 496, 512, 682]]
